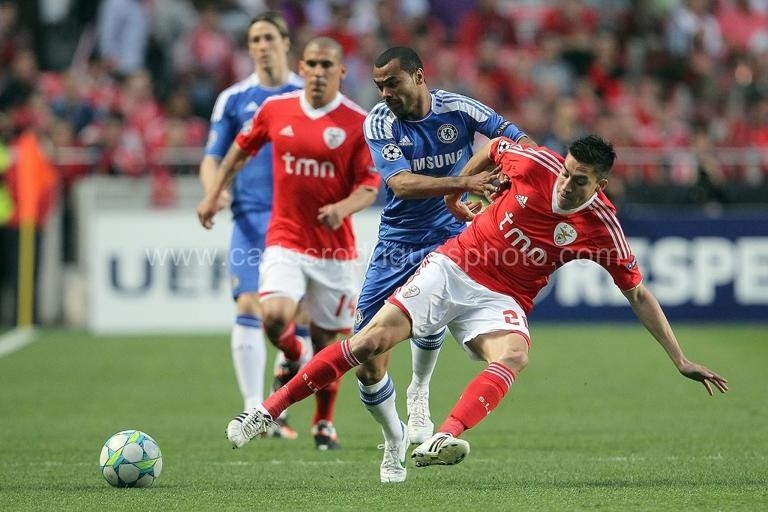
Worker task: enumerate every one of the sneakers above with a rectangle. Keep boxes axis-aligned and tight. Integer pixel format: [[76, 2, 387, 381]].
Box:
[[377, 390, 470, 483], [224, 335, 341, 450]]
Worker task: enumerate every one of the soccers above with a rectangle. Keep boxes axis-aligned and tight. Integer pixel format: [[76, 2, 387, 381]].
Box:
[[100, 430, 162, 487]]
